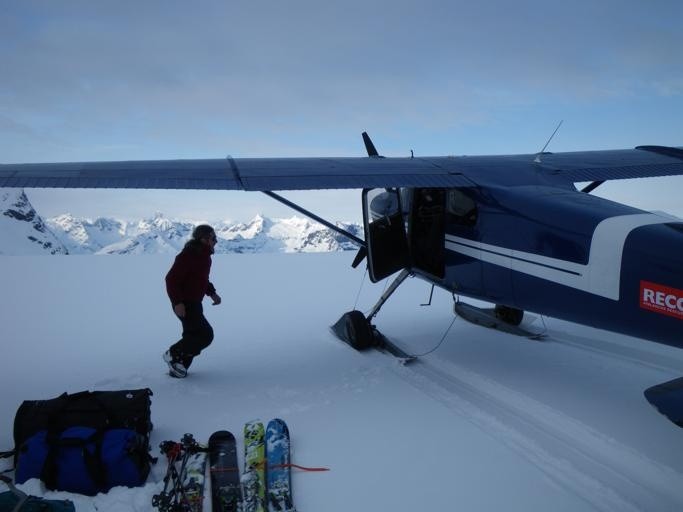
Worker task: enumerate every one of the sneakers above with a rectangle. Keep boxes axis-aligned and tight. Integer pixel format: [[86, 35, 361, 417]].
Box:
[[163, 350, 186, 378]]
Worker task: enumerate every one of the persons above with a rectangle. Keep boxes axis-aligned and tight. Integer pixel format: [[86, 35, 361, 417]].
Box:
[[162, 224, 222, 379]]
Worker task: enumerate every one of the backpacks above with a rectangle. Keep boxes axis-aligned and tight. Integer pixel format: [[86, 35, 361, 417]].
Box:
[[13, 388, 150, 446], [0, 491, 75, 512], [16, 426, 149, 495]]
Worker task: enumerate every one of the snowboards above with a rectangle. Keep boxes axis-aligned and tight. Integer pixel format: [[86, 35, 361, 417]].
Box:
[[242, 419, 265, 512], [265, 418, 294, 512], [180, 442, 206, 512], [208, 430, 241, 512]]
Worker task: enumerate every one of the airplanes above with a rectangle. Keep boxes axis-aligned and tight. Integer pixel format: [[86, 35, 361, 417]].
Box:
[[0, 124, 681, 430]]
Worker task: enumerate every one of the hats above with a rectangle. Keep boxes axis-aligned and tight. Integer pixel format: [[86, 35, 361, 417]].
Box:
[[193, 225, 213, 238]]
